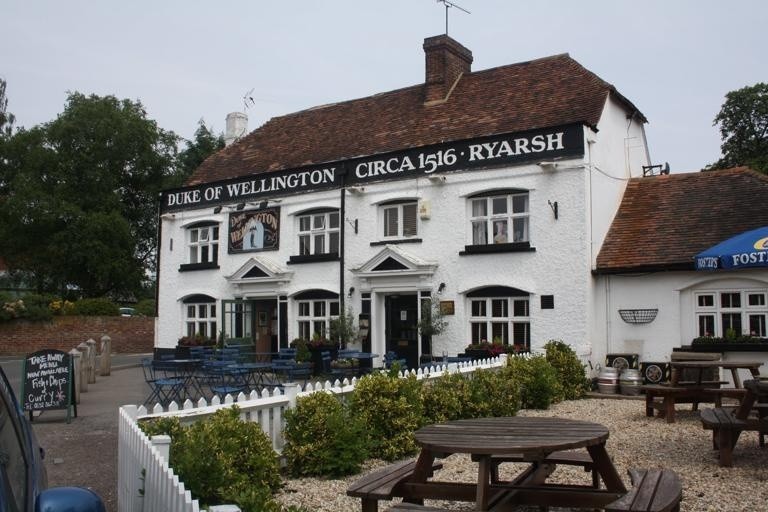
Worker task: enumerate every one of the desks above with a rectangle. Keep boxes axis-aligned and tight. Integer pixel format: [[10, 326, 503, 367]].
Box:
[[740, 378, 768, 395], [411, 416, 609, 512], [661, 360, 765, 423]]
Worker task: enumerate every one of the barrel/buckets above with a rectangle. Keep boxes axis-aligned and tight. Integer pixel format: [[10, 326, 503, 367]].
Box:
[[598, 366, 619, 393], [619, 369, 643, 396]]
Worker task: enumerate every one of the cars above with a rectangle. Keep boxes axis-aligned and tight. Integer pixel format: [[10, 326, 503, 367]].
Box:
[[0, 365, 105, 511], [118, 307, 146, 318]]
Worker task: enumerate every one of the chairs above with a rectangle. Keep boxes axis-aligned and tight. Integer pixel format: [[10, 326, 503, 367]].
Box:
[[140, 341, 408, 408]]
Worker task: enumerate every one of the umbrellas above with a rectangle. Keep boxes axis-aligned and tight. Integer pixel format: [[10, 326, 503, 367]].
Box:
[[693, 226, 768, 274]]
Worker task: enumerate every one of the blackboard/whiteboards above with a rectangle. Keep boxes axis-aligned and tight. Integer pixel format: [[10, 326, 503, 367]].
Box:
[[23, 348, 70, 411]]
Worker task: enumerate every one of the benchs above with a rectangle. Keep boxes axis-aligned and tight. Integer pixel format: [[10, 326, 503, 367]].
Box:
[[695, 403, 749, 460], [635, 377, 748, 425], [602, 466, 684, 512], [383, 501, 469, 512], [488, 452, 601, 489], [345, 458, 444, 511]]
